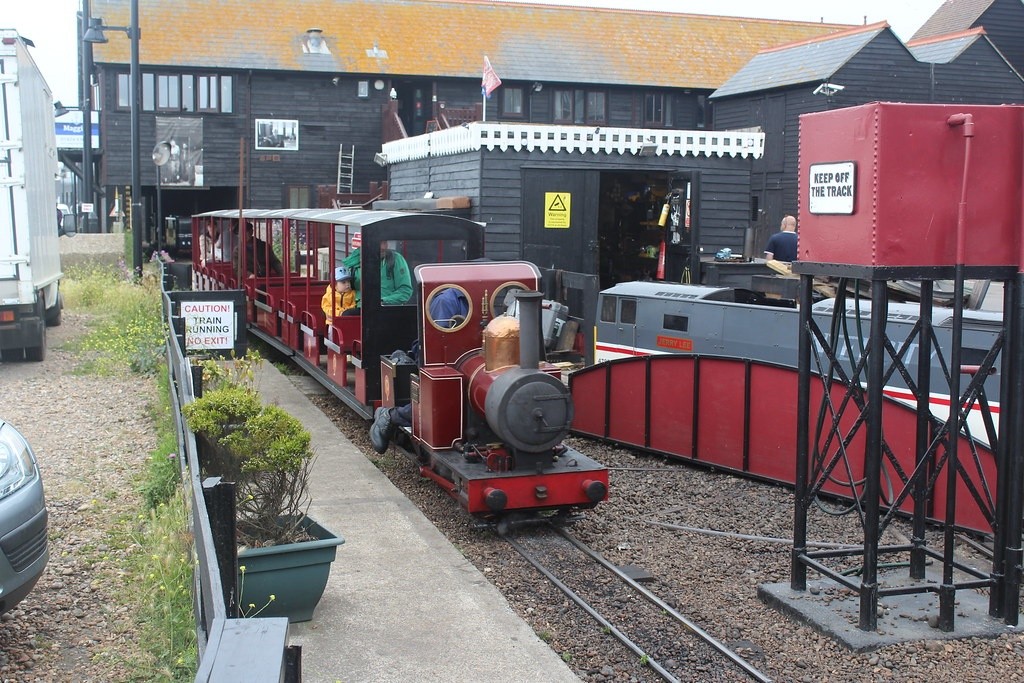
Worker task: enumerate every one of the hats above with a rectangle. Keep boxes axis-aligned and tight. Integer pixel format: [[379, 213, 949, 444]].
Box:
[[335, 267, 353, 281], [233, 222, 253, 233]]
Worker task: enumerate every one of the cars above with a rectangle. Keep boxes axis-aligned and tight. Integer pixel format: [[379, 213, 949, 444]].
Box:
[[0, 415, 51, 619]]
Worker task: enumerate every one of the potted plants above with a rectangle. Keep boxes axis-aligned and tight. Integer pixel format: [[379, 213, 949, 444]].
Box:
[[217, 395, 345, 622]]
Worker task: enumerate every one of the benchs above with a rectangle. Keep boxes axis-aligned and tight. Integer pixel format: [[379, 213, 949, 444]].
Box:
[[197, 261, 366, 407]]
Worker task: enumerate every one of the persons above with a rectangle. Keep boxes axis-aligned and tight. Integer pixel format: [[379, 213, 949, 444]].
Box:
[[763, 216, 798, 299], [200, 220, 284, 307], [320, 241, 414, 325], [170, 140, 205, 186], [368, 287, 470, 455]]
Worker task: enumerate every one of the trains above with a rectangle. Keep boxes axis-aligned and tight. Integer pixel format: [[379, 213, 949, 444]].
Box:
[[193, 207, 610, 537]]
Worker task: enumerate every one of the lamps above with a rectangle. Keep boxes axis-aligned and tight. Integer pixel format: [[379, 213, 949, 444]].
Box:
[[639, 143, 658, 158]]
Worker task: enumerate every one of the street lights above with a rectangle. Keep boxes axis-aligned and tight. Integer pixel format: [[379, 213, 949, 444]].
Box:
[[82, 0, 144, 279]]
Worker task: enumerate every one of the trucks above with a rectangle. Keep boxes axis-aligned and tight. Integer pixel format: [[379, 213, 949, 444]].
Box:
[[0, 27, 64, 364]]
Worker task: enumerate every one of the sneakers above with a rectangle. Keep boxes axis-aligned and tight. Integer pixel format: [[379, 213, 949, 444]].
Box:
[[369, 407, 395, 455]]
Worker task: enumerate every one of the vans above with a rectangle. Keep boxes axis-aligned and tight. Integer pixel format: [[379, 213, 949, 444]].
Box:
[[56, 202, 70, 229]]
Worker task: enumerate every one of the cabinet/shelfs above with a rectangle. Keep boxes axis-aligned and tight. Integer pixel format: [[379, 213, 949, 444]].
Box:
[[615, 178, 665, 282]]
